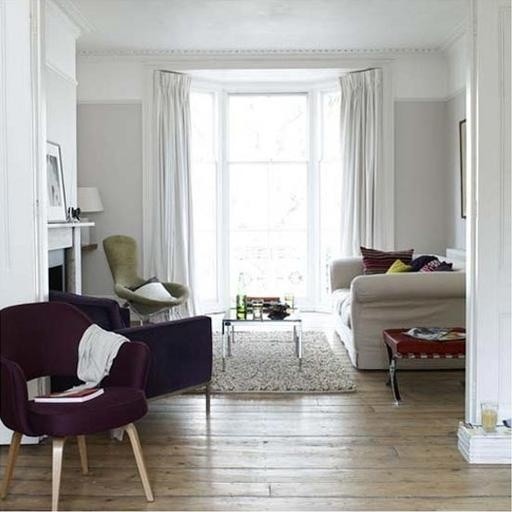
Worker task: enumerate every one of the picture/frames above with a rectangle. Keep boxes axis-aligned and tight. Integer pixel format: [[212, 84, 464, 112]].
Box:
[[46, 140, 69, 223]]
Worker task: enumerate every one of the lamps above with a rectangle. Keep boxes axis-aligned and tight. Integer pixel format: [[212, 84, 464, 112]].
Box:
[[77, 187, 104, 245]]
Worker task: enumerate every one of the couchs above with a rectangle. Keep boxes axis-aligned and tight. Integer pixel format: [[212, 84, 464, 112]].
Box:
[[329, 253, 465, 370]]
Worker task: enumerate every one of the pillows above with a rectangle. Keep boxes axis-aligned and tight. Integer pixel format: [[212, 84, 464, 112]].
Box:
[[360, 246, 453, 274], [132, 276, 175, 300]]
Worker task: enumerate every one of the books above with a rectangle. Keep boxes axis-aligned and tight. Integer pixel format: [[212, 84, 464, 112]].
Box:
[[402, 324, 457, 344], [456, 417, 512, 468], [33, 385, 104, 404], [245, 296, 280, 302], [438, 330, 466, 342], [244, 303, 282, 308]]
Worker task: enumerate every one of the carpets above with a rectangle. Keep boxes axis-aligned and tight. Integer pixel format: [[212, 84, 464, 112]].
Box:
[[186, 329, 356, 394]]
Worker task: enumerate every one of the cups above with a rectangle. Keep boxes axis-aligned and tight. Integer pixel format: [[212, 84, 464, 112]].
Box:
[[252, 303, 263, 319], [285, 295, 294, 309], [480, 401, 500, 434], [236, 294, 247, 313]]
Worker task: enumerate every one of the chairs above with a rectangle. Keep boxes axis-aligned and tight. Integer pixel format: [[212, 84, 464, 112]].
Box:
[[49, 289, 212, 441], [102, 236, 190, 324], [0, 301, 155, 512]]
